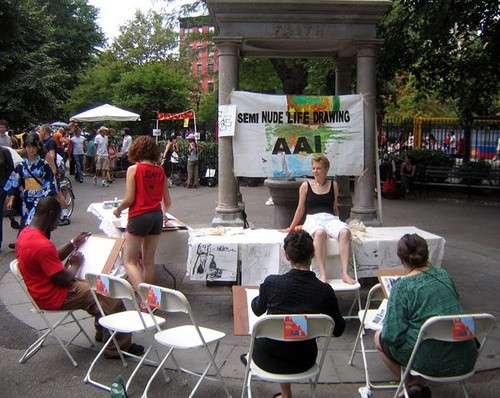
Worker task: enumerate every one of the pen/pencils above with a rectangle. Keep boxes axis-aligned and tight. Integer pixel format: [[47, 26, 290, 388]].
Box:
[[68, 251, 77, 260]]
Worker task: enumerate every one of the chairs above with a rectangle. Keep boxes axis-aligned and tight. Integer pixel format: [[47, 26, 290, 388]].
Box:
[[347, 283, 399, 389], [83, 273, 169, 393], [10, 259, 94, 367], [393, 313, 497, 398], [241, 314, 336, 398], [138, 283, 233, 398]]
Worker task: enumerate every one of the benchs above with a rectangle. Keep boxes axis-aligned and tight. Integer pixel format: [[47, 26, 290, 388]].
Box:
[[396, 165, 500, 202]]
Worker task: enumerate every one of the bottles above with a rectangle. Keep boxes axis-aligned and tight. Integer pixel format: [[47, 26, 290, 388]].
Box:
[[111, 376, 127, 398], [113, 197, 119, 208]]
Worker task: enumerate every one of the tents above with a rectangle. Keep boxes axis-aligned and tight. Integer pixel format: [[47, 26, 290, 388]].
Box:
[[72, 103, 142, 129]]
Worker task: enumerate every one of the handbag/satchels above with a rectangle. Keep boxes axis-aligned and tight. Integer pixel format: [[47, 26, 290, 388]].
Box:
[[189, 149, 201, 165], [57, 164, 65, 180], [382, 178, 395, 192], [171, 151, 179, 163], [108, 145, 114, 154], [55, 192, 66, 209]]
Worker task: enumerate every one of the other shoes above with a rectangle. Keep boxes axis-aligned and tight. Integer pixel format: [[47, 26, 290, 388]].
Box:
[[186, 184, 190, 188], [75, 177, 79, 181], [104, 343, 145, 360], [194, 185, 198, 188], [102, 180, 109, 187], [107, 180, 112, 184], [138, 300, 147, 313], [93, 176, 97, 184], [273, 393, 281, 398], [397, 384, 432, 398], [95, 333, 103, 342], [80, 179, 83, 183], [83, 172, 86, 176], [240, 354, 247, 365], [8, 243, 15, 249]]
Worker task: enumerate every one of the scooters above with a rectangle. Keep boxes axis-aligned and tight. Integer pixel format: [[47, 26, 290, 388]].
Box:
[[168, 153, 219, 188]]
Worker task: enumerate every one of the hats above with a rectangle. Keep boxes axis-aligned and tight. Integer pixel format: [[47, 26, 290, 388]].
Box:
[[185, 134, 195, 140], [98, 126, 109, 132]]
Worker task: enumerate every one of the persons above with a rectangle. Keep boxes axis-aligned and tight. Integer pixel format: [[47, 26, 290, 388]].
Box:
[[374, 234, 481, 398], [0, 120, 206, 357], [281, 153, 359, 285], [240, 229, 347, 398], [376, 130, 500, 200]]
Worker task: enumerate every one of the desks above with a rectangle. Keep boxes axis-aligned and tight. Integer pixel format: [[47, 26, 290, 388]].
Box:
[[185, 226, 445, 286], [86, 203, 193, 289]]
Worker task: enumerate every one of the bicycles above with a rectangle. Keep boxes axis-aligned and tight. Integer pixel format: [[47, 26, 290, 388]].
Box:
[[56, 177, 76, 222]]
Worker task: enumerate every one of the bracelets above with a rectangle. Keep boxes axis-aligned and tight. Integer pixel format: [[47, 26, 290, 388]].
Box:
[[71, 238, 77, 248]]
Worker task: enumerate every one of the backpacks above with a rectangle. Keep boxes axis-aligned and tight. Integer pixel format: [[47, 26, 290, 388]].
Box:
[[380, 159, 393, 181]]
